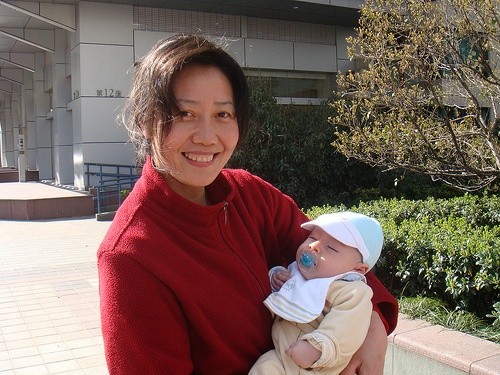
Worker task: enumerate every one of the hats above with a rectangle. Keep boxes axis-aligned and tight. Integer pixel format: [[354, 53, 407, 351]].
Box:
[[300, 212, 384, 274]]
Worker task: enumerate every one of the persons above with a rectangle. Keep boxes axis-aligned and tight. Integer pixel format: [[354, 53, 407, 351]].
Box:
[[97, 33, 398, 375], [248, 212, 384, 375]]
